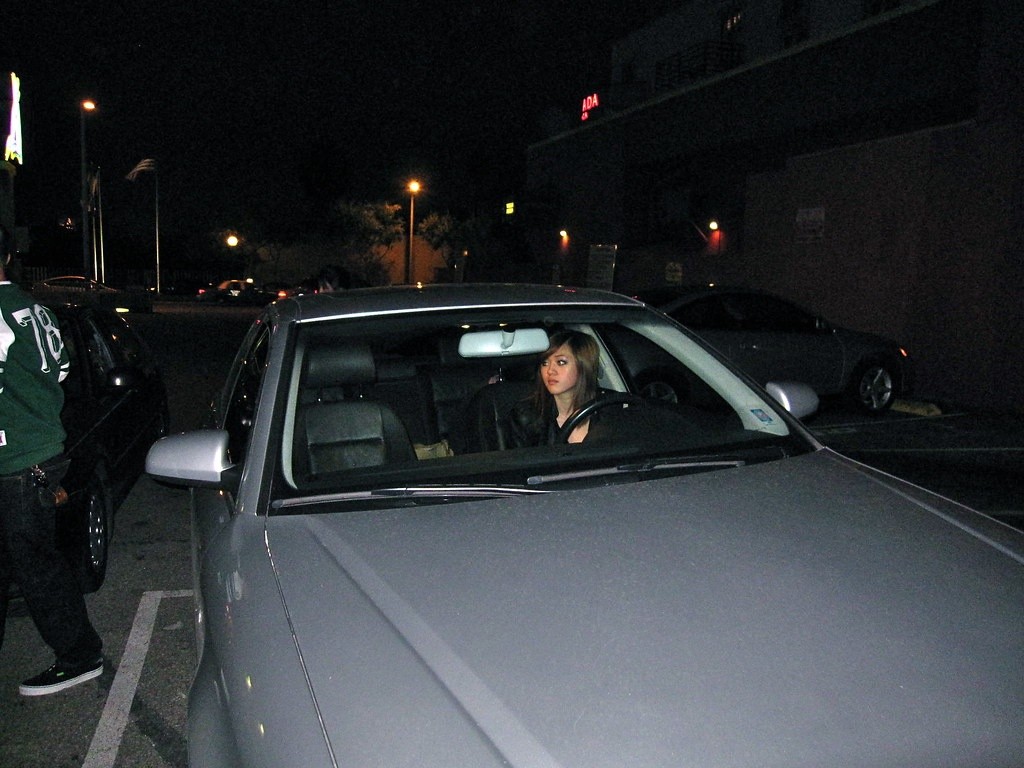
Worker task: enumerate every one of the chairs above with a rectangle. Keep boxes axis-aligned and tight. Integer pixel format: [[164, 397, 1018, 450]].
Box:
[[483, 353, 547, 454], [301, 343, 418, 476]]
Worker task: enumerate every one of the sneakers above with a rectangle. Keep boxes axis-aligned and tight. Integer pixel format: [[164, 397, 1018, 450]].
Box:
[[19, 657, 104, 697]]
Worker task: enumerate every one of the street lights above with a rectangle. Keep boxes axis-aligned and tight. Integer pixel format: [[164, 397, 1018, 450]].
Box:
[[407, 179, 422, 284], [79, 98, 100, 296], [226, 236, 238, 279]]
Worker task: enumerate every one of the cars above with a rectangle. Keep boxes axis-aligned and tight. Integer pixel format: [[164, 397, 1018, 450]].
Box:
[[593, 280, 912, 416], [32, 276, 150, 314], [143, 280, 1024, 768], [40, 290, 171, 596], [195, 281, 316, 307], [146, 281, 175, 295]]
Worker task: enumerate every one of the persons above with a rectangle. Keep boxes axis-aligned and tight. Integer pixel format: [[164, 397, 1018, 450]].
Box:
[[505, 330, 637, 450], [0, 225, 105, 696]]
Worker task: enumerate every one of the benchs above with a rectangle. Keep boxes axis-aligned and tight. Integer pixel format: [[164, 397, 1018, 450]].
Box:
[[370, 330, 490, 457]]
